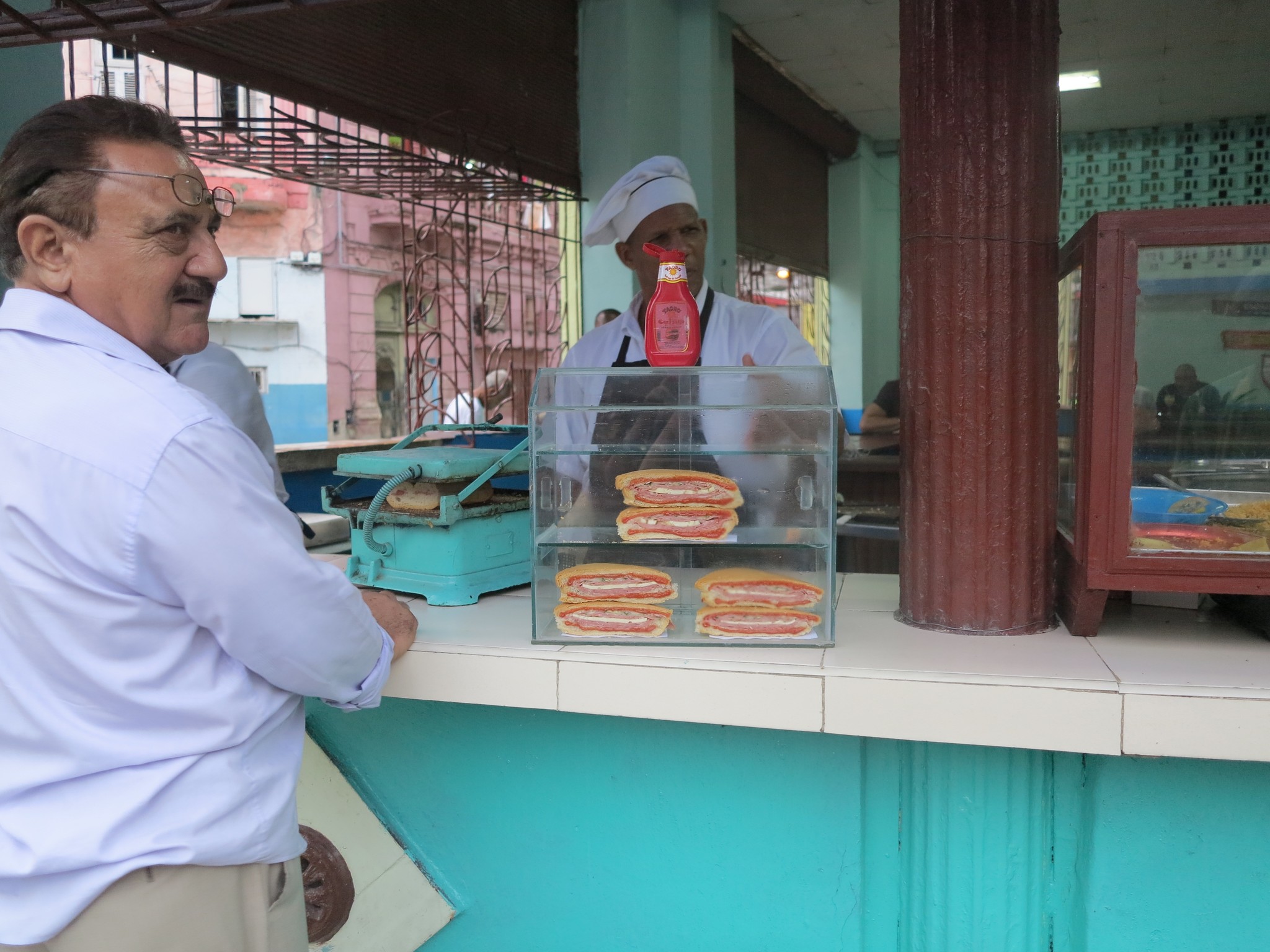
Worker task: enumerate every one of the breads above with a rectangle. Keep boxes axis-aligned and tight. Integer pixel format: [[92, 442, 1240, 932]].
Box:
[[387, 475, 492, 510]]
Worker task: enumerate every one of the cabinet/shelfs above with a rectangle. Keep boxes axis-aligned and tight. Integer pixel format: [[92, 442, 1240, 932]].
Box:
[[528, 368, 840, 648]]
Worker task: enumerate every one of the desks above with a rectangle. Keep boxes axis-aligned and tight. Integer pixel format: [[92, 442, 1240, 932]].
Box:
[[846, 434, 899, 453]]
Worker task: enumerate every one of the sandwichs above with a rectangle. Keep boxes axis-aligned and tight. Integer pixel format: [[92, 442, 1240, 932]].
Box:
[[553, 562, 679, 638], [695, 567, 825, 637], [616, 469, 745, 541]]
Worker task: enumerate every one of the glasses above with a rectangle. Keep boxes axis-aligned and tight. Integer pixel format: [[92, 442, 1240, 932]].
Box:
[[25, 167, 236, 218]]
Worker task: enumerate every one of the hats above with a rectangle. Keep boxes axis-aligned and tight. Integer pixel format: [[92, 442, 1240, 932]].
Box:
[[581, 154, 700, 248]]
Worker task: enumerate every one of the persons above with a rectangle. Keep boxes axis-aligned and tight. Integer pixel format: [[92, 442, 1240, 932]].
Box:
[[1133, 363, 1222, 412], [0, 93, 624, 952], [550, 155, 901, 572]]
